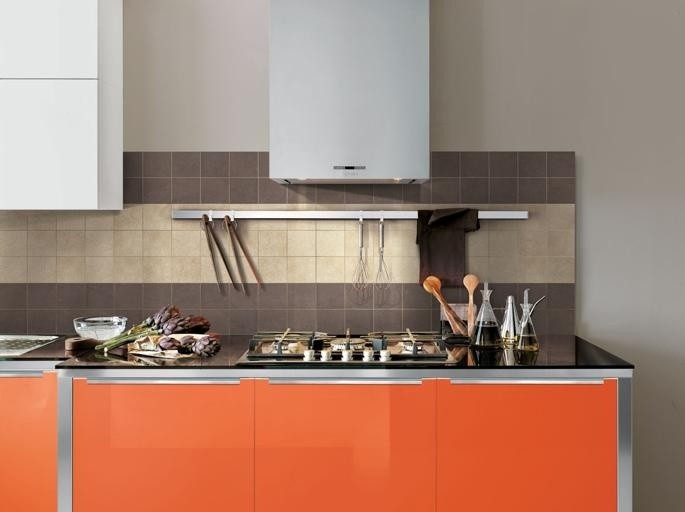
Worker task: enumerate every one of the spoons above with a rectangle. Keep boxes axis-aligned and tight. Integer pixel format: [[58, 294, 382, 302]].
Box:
[[423, 276, 467, 335], [464, 274, 480, 336]]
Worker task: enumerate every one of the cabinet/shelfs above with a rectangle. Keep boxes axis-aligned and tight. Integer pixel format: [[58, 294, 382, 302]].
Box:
[[0, 371, 58, 512], [72, 378, 254, 512], [254, 377, 436, 512], [2, 1, 123, 211], [435, 378, 618, 511]]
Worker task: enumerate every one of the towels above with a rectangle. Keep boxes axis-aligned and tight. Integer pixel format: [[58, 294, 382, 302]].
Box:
[[415, 206, 480, 287]]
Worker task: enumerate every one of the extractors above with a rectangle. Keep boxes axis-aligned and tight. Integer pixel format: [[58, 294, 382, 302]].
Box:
[[268, 0, 429, 184]]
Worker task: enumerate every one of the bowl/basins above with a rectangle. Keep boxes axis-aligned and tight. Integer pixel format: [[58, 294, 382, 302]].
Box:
[[73, 315, 128, 340]]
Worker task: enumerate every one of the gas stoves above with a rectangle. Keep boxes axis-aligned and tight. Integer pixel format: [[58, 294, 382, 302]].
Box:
[[236, 328, 458, 367]]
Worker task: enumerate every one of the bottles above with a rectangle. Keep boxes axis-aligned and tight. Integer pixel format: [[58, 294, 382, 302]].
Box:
[[515, 289, 544, 352], [471, 281, 503, 346]]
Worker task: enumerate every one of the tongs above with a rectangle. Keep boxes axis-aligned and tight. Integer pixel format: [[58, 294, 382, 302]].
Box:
[[202, 215, 236, 294], [225, 215, 262, 294]]
